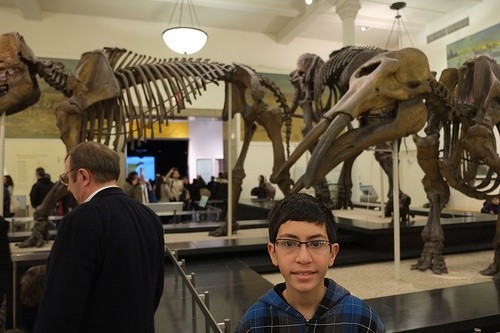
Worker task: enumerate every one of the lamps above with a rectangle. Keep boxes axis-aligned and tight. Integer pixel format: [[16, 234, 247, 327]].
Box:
[[161, 0, 208, 55]]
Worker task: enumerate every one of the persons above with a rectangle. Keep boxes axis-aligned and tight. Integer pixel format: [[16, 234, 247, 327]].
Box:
[[3, 175, 15, 217], [124, 171, 142, 202], [184, 172, 228, 221], [153, 175, 174, 202], [140, 176, 156, 203], [232, 194, 385, 333], [34, 141, 164, 333], [163, 167, 183, 202], [32, 168, 77, 216], [250, 175, 268, 198], [484, 198, 500, 214]]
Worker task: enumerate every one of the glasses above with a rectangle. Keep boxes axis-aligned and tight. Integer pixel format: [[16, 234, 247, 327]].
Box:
[[58, 167, 79, 186], [273, 239, 333, 255]]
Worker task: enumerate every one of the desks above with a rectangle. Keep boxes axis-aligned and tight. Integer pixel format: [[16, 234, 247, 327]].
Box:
[[9, 227, 269, 333]]
